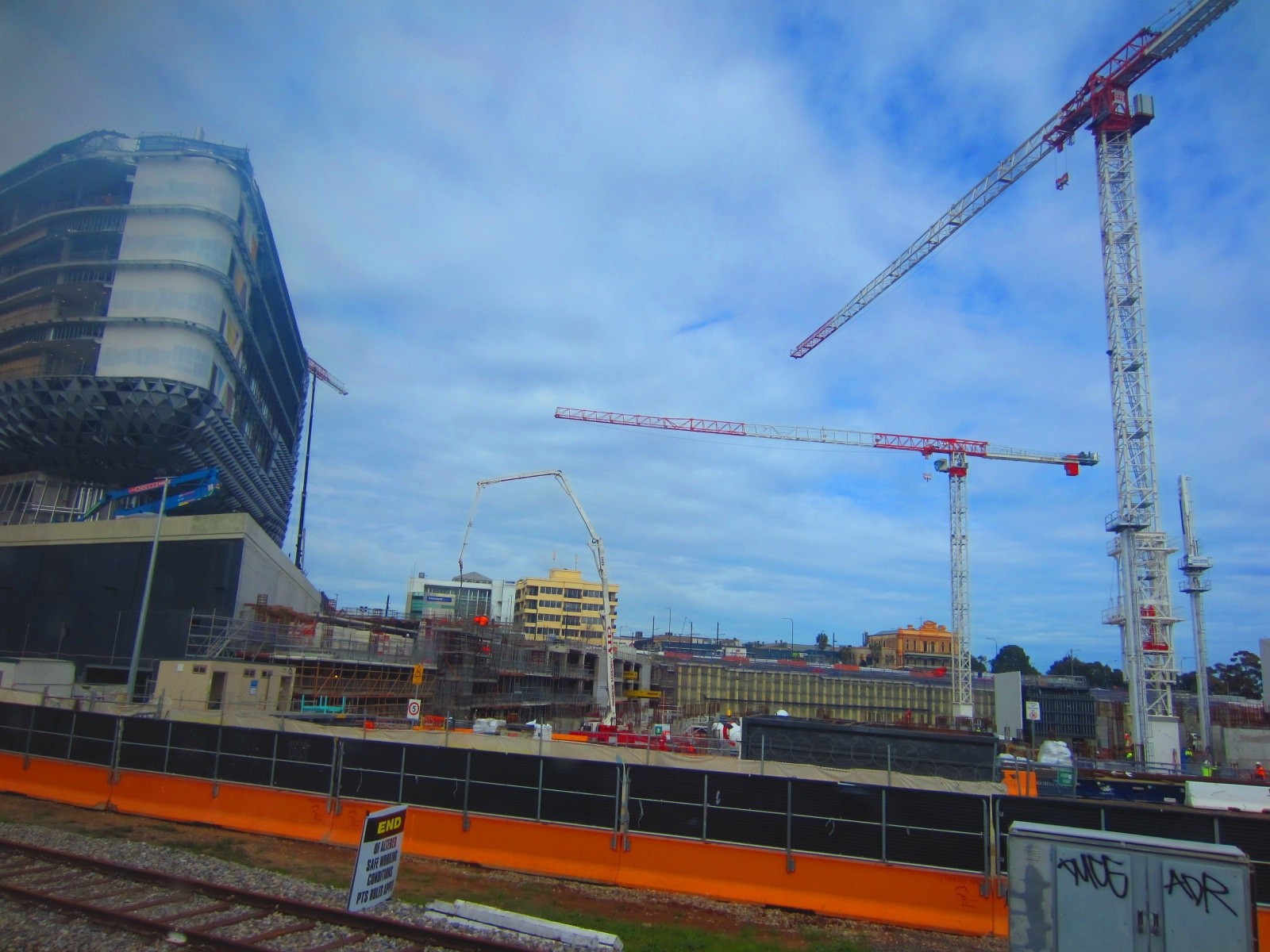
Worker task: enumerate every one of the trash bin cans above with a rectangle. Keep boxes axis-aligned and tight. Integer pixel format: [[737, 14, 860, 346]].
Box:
[[444, 718, 455, 731]]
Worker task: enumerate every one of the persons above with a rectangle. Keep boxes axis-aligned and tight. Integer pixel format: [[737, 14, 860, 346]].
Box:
[[1127, 752, 1132, 758], [1202, 760, 1266, 778], [1185, 748, 1192, 759]]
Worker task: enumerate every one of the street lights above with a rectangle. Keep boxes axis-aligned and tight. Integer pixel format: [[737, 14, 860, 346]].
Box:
[[666, 607, 670, 653], [985, 638, 998, 657], [781, 617, 793, 660]]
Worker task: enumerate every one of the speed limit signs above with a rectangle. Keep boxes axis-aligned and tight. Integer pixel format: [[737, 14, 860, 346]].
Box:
[[407, 698, 421, 722]]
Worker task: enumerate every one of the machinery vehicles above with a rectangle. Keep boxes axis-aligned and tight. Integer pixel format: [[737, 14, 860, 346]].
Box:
[[453, 470, 693, 756]]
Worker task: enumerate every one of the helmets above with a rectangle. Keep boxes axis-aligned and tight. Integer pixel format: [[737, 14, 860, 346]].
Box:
[[1255, 761, 1261, 765], [1204, 760, 1211, 764]]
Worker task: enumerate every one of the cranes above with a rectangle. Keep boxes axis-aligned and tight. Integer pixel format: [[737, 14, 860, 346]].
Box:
[[554, 400, 1100, 735], [791, 1, 1249, 803], [305, 357, 348, 396]]
